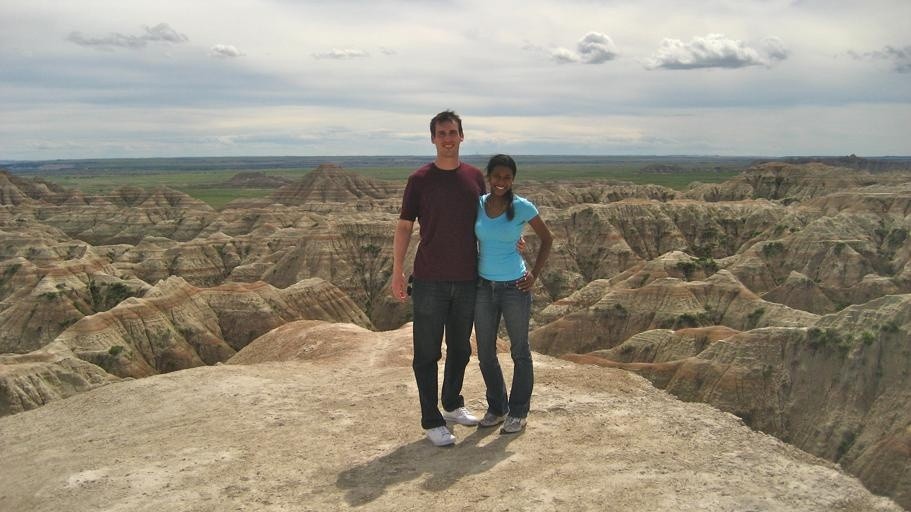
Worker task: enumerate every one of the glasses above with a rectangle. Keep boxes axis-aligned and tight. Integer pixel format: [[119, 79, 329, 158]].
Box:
[[404, 272, 413, 298]]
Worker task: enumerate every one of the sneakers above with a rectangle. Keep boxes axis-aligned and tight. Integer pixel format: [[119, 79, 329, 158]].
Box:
[[479, 410, 509, 427], [423, 425, 456, 446], [501, 415, 527, 433], [442, 406, 479, 425]]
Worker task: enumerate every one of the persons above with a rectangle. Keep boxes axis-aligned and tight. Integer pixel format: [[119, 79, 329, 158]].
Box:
[[474, 154, 553, 433], [392, 110, 526, 447]]
